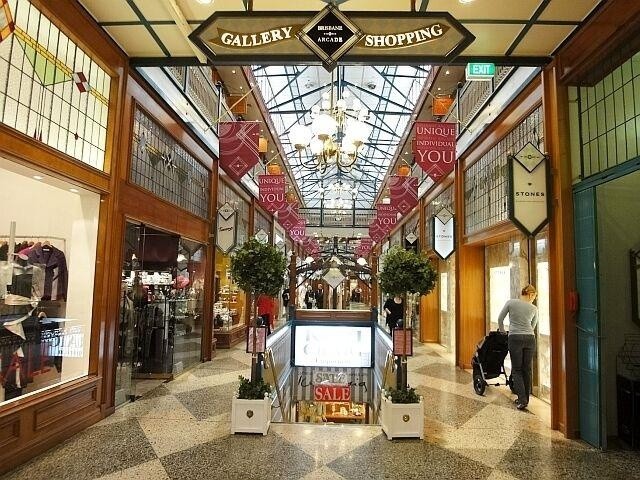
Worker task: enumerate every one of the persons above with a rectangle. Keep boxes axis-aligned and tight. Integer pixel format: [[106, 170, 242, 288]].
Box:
[[258, 291, 273, 336], [383, 293, 404, 336], [282, 282, 364, 322], [496, 284, 539, 410]]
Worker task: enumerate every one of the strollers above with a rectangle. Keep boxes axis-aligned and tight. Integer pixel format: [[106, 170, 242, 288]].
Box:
[[470, 328, 519, 395]]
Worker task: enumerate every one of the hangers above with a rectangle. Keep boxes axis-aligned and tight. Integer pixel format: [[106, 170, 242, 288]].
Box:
[[0, 303, 58, 328], [0, 236, 64, 254]]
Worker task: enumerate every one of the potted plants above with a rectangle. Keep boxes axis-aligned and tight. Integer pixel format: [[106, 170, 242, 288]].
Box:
[[222, 238, 287, 435], [377, 244, 438, 442]]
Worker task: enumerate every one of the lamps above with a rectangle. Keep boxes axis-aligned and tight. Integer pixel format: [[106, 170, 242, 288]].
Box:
[[290, 67, 373, 221]]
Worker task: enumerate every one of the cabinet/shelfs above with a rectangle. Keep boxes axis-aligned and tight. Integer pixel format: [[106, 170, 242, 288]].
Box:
[[118, 268, 176, 378]]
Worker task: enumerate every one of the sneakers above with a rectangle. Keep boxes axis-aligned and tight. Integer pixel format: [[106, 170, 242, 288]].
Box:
[[515, 398, 529, 409]]
